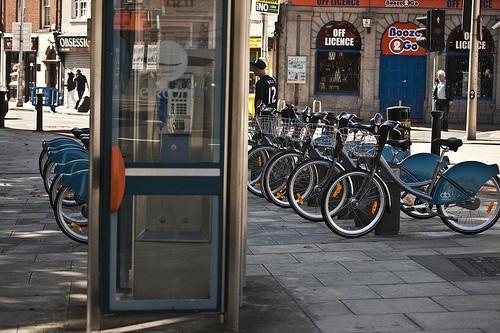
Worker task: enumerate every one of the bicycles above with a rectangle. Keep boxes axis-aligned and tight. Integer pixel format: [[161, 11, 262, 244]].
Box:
[[39, 127, 90, 245], [246, 103, 500, 238]]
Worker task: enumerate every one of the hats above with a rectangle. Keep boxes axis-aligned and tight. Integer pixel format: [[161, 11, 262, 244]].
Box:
[[250, 60, 267, 70]]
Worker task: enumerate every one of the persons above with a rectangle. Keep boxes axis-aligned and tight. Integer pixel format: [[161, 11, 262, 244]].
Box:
[[432, 69, 454, 132], [250, 59, 277, 123], [64, 72, 77, 108], [74, 69, 89, 100]]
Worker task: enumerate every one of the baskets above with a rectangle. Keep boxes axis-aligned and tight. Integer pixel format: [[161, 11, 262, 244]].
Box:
[[253, 113, 380, 157]]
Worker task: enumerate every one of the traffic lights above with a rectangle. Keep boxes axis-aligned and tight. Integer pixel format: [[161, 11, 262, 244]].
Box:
[[415, 8, 445, 52]]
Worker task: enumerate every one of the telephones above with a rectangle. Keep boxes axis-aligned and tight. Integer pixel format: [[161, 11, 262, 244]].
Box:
[[156, 73, 194, 134]]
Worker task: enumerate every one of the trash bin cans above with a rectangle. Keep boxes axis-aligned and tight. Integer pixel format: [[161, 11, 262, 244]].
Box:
[[387, 106, 411, 155]]
